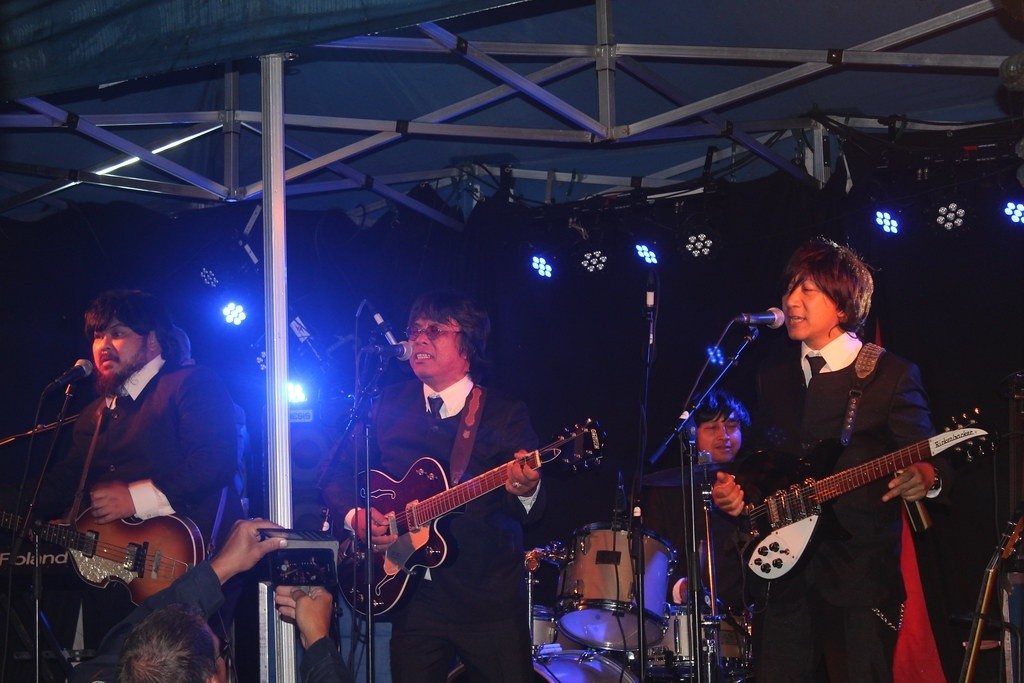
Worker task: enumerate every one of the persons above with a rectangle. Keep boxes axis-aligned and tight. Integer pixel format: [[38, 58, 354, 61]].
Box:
[[23, 289, 247, 683], [711, 238, 953, 683], [69, 518, 352, 683], [316, 291, 559, 683], [641, 390, 765, 604]]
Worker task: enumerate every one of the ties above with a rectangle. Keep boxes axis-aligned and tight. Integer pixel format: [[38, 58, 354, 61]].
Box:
[[426, 395, 445, 422], [804, 354, 826, 390]]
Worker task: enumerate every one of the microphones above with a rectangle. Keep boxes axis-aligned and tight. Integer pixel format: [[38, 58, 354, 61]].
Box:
[[42, 359, 93, 394], [362, 341, 413, 361], [732, 307, 785, 329], [363, 301, 398, 346], [646, 278, 654, 345], [614, 474, 628, 520]]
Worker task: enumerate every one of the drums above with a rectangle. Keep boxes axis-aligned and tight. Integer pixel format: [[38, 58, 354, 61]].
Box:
[[535, 648, 641, 683], [554, 519, 679, 651], [645, 603, 754, 676], [531, 602, 557, 656]]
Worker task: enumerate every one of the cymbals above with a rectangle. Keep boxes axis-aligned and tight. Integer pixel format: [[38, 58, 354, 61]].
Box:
[[642, 461, 737, 488]]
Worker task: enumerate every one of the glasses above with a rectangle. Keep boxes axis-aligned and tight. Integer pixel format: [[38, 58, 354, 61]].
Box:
[[209, 633, 232, 666], [403, 324, 462, 341]]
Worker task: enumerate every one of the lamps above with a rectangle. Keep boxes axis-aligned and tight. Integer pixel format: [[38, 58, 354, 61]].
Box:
[[869, 180, 934, 241], [927, 171, 981, 240], [569, 216, 619, 280], [524, 229, 567, 282], [197, 231, 256, 290], [672, 194, 740, 264]]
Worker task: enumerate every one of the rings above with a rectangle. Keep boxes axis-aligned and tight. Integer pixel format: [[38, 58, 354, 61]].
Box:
[[512, 482, 521, 488], [372, 544, 377, 550]]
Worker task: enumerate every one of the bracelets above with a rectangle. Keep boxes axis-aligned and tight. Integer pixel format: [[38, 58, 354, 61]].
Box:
[[929, 467, 941, 491]]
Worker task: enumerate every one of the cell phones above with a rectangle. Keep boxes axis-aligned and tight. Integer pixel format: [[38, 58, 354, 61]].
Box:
[[270, 548, 337, 587]]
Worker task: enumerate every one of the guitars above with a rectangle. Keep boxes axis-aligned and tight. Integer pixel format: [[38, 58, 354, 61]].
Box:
[[959, 497, 1024, 683], [700, 406, 996, 607], [0, 505, 205, 608], [335, 418, 606, 616]]
